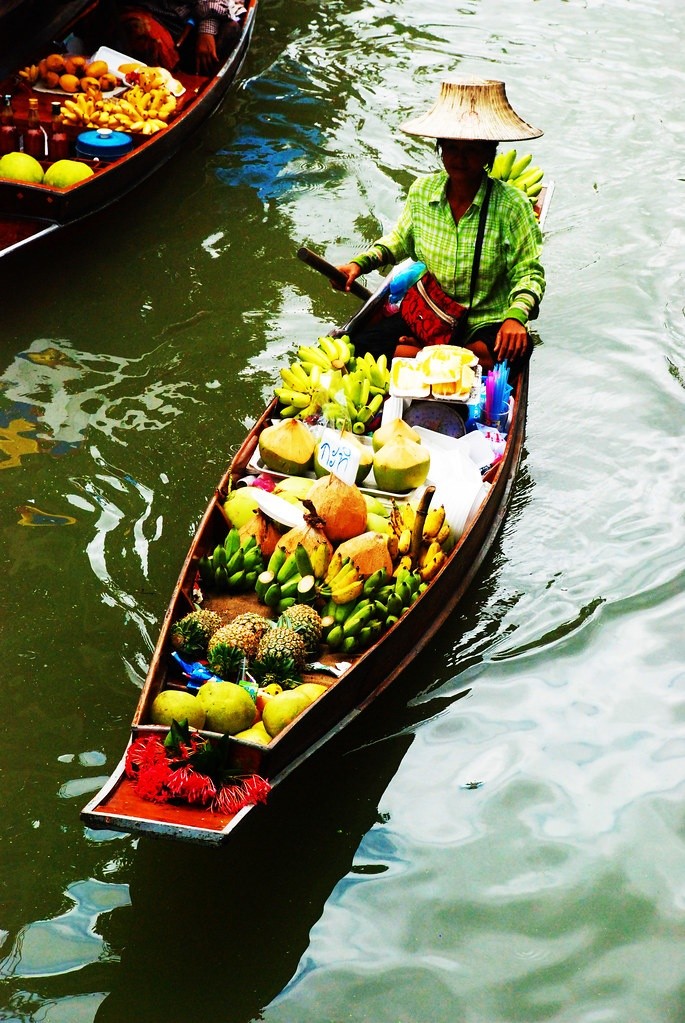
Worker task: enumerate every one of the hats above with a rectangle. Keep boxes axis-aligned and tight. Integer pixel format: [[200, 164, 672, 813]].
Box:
[[399, 77, 545, 141]]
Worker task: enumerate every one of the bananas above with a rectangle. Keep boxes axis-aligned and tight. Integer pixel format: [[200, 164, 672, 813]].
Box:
[[199, 500, 451, 653], [18, 64, 39, 83], [273, 333, 392, 435], [58, 70, 178, 135], [485, 148, 544, 205]]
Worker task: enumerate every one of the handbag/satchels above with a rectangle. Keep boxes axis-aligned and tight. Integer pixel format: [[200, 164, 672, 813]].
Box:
[[400, 271, 467, 345]]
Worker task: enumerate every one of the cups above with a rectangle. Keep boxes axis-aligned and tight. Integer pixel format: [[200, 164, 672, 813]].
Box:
[[479, 400, 510, 430]]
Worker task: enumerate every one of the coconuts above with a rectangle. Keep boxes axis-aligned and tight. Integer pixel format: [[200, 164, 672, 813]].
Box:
[[224, 417, 430, 574], [150, 680, 328, 746], [0, 151, 94, 189]]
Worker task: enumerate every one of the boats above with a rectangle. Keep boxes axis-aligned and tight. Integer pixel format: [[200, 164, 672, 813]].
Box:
[[0, 0, 259, 265], [80, 175, 560, 850]]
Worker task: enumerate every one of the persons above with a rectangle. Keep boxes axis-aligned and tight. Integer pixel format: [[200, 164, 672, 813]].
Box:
[[100, 0, 247, 75], [330, 80, 546, 372]]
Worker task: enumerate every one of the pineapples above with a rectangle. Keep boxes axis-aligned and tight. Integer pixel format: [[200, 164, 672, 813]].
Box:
[[391, 344, 478, 396], [170, 603, 321, 682]]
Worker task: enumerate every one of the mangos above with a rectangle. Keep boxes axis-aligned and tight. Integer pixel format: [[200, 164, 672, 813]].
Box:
[[40, 55, 117, 93]]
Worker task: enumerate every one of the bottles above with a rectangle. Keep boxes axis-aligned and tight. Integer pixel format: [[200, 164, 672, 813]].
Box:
[[46, 101, 68, 163], [75, 127, 134, 162], [23, 98, 45, 161], [0, 95, 18, 156]]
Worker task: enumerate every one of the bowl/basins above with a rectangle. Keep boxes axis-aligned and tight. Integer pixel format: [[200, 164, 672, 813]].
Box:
[[91, 46, 145, 78]]
